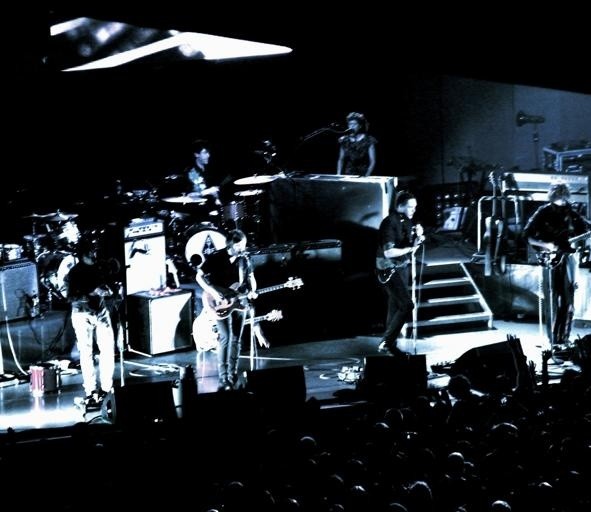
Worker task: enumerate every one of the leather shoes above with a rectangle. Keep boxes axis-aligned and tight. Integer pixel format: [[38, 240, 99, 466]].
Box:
[[377, 346, 404, 356]]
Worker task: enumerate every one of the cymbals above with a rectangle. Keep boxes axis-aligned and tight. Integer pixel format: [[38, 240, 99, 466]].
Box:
[[233, 174, 279, 186], [234, 189, 263, 197], [23, 213, 55, 221], [50, 212, 79, 222], [24, 233, 46, 241]]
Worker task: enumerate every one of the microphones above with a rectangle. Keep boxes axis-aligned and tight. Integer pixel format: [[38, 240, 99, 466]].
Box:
[[344, 128, 354, 135]]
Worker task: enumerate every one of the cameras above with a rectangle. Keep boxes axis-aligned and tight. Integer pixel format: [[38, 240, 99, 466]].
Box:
[[500, 396, 508, 405]]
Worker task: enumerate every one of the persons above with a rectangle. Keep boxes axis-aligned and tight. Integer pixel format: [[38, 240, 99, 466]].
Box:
[[376, 190, 426, 356], [181, 142, 211, 194], [58, 364, 590, 510], [195, 231, 259, 389], [56, 238, 116, 410], [523, 182, 591, 350], [337, 113, 377, 176]]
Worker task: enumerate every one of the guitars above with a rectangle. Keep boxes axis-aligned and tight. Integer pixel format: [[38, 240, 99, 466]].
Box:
[[482, 172, 507, 276], [203, 276, 303, 321], [191, 309, 283, 351], [533, 227, 590, 270]]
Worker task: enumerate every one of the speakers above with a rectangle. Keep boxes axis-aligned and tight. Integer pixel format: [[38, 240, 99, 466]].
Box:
[[363, 355, 427, 398], [113, 379, 174, 426], [248, 366, 306, 402], [451, 338, 523, 393]]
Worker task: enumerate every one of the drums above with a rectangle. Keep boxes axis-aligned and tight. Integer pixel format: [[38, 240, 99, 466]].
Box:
[[38, 248, 80, 299], [162, 196, 208, 241], [219, 201, 249, 223], [176, 222, 229, 273]]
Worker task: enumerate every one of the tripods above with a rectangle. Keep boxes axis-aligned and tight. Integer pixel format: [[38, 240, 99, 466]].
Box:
[[234, 303, 258, 374]]
